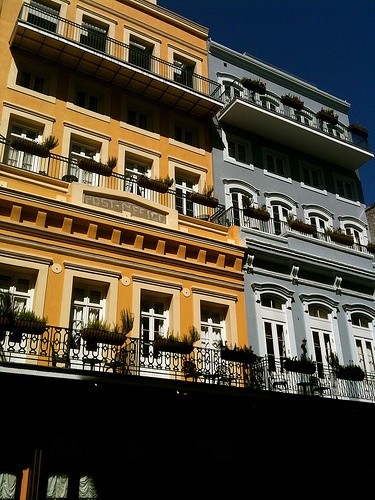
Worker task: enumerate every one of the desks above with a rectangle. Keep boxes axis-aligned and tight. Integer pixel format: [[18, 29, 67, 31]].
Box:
[[82, 360, 103, 371], [297, 382, 310, 394]]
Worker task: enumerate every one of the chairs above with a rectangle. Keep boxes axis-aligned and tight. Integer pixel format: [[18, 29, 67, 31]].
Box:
[[268, 369, 289, 391], [183, 361, 202, 382], [52, 345, 70, 369], [107, 352, 125, 373], [309, 376, 332, 397]]
